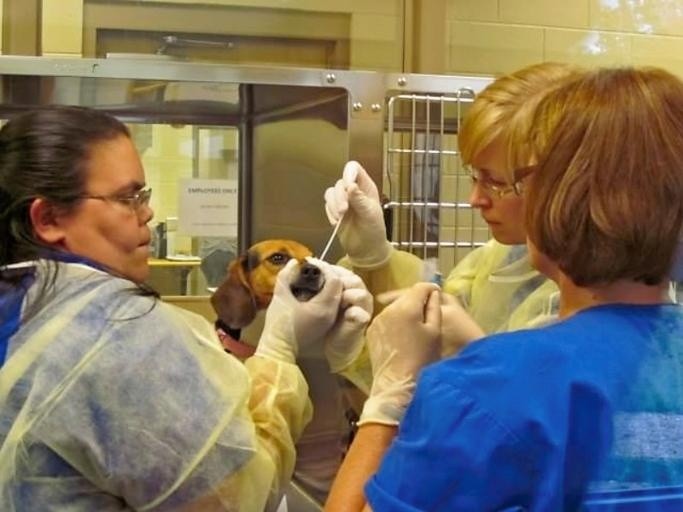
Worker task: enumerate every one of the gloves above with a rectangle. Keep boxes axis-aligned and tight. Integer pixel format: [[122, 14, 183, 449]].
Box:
[[356, 281, 442, 426], [376, 288, 486, 361], [324, 160, 392, 271], [253, 256, 343, 366], [324, 264, 374, 375]]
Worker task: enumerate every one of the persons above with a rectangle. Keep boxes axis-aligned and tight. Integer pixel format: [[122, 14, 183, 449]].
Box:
[[326, 66, 683, 512], [323, 63, 584, 394], [1, 103, 343, 512]]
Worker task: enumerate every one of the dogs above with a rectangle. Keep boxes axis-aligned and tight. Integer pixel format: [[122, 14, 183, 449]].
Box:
[[210, 238, 325, 365]]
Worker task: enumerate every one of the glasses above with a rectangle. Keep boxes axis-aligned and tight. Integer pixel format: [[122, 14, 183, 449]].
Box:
[[513, 164, 537, 197], [75, 188, 152, 211], [464, 165, 513, 200]]
